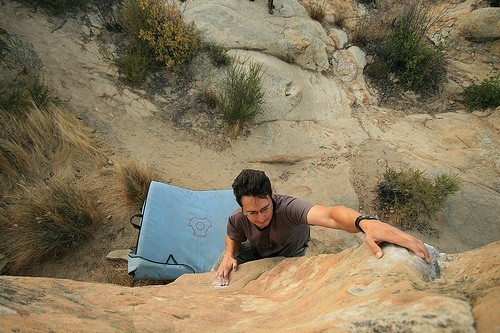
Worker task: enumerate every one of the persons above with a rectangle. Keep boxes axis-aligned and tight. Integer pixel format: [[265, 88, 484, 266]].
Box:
[[211, 169, 432, 286]]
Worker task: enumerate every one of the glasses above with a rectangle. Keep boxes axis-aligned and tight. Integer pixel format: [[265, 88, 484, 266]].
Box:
[[243, 203, 273, 217]]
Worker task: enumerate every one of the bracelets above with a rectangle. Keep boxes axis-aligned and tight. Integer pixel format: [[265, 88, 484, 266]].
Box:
[[355, 215, 378, 234]]
